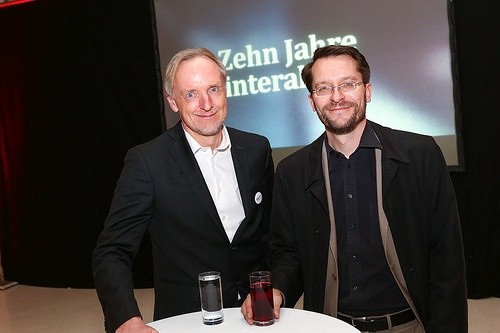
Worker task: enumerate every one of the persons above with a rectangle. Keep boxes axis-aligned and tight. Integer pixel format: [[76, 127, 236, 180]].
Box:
[[92, 47, 275, 333], [241, 45, 469, 333]]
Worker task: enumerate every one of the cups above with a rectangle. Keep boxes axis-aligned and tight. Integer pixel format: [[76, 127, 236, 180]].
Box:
[[249, 271, 275, 326], [199, 272, 223, 325]]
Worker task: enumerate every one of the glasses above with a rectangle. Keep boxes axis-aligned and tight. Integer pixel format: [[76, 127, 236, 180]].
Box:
[[311, 80, 365, 95]]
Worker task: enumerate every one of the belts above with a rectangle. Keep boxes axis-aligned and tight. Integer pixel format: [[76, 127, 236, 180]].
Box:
[[336, 309, 416, 333]]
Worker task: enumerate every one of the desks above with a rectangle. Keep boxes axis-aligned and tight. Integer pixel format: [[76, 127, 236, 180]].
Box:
[[145, 308, 362, 333]]
